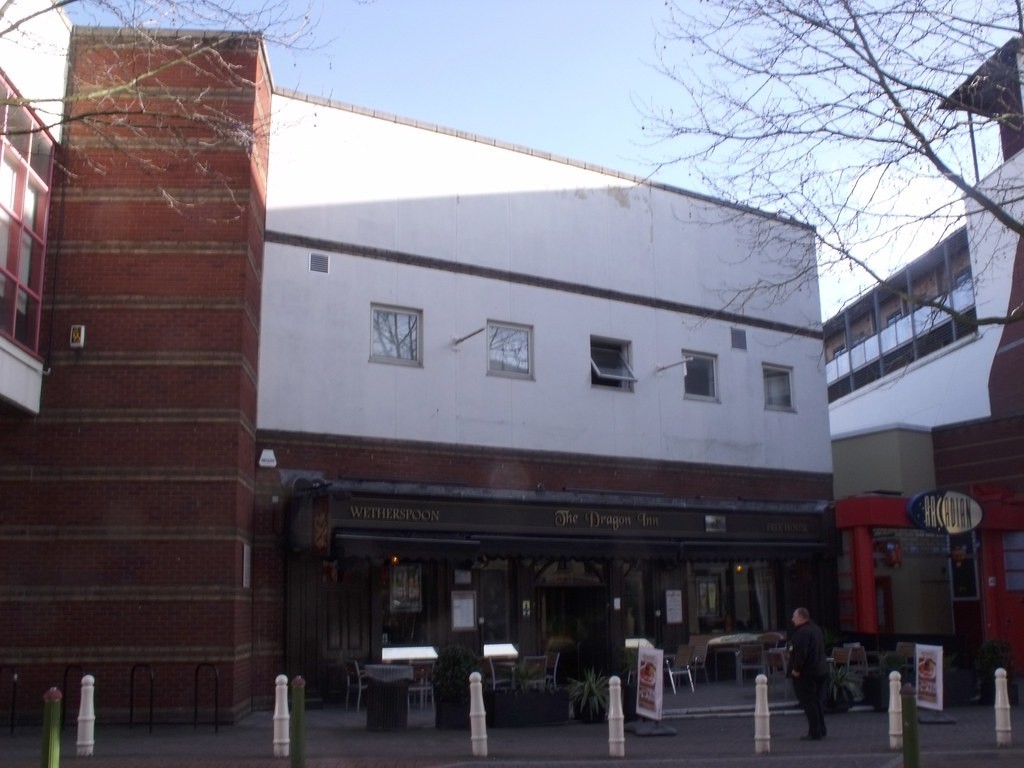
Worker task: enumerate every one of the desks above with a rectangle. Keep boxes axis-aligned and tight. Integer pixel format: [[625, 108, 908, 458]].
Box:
[[866, 650, 899, 668]]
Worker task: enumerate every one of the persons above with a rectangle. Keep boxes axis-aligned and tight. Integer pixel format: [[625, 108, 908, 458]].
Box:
[[786, 608, 827, 740]]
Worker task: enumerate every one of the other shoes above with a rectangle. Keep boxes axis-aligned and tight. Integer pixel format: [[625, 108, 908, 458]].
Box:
[[800, 734, 822, 740]]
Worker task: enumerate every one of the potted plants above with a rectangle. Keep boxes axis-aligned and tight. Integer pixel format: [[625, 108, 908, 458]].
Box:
[[566, 666, 610, 723], [435, 656, 469, 727], [820, 664, 860, 714], [974, 637, 1019, 708]]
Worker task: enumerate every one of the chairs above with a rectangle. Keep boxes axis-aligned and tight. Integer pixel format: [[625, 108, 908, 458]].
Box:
[[625, 638, 645, 684], [544, 652, 560, 689], [850, 647, 880, 677], [519, 655, 548, 692], [831, 647, 852, 671], [344, 660, 371, 713], [408, 659, 437, 713], [678, 640, 710, 688], [739, 644, 765, 686], [713, 648, 741, 683], [663, 659, 695, 694], [770, 649, 796, 699], [844, 642, 863, 672], [895, 642, 916, 678], [481, 656, 517, 692]]
